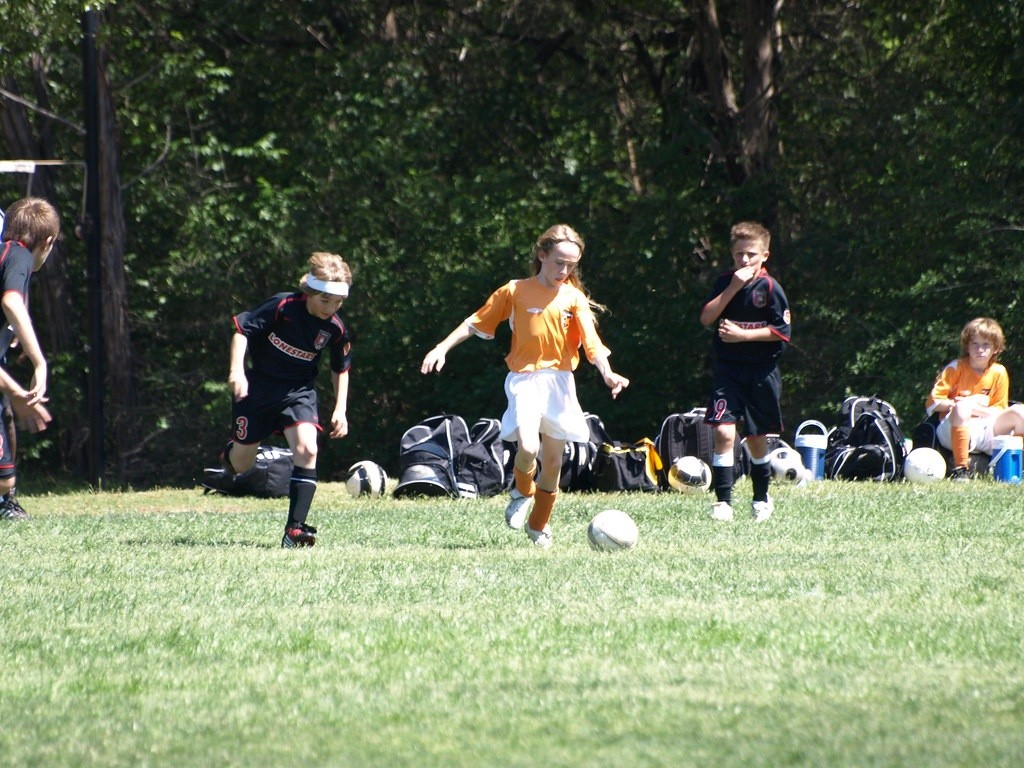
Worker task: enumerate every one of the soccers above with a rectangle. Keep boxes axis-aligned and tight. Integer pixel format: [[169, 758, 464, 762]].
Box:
[[668, 454, 714, 494], [903, 447, 948, 483], [345, 460, 388, 498], [587, 510, 638, 552], [769, 448, 805, 486]]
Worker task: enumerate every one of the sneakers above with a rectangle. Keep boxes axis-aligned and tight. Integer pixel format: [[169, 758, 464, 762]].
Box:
[[525, 520, 552, 548], [711, 501, 733, 522], [948, 466, 970, 482], [282, 527, 316, 548], [223, 441, 246, 496], [505, 487, 532, 529], [749, 493, 774, 522], [0, 489, 33, 521]]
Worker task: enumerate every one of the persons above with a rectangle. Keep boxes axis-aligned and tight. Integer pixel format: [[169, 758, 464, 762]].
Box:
[[421, 224, 629, 551], [0, 196, 61, 522], [699, 219, 791, 526], [925, 317, 1024, 483], [219, 252, 353, 548]]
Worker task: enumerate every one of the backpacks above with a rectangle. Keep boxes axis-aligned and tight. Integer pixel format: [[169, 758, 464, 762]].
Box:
[[824, 393, 906, 482], [399, 408, 744, 498], [913, 410, 992, 478]]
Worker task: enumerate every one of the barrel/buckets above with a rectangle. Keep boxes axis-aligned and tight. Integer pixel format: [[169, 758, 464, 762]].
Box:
[[988, 435, 1023, 484], [794, 420, 828, 480]]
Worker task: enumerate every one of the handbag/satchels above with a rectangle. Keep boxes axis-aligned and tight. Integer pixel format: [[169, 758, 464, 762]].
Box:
[[202, 442, 296, 498]]
